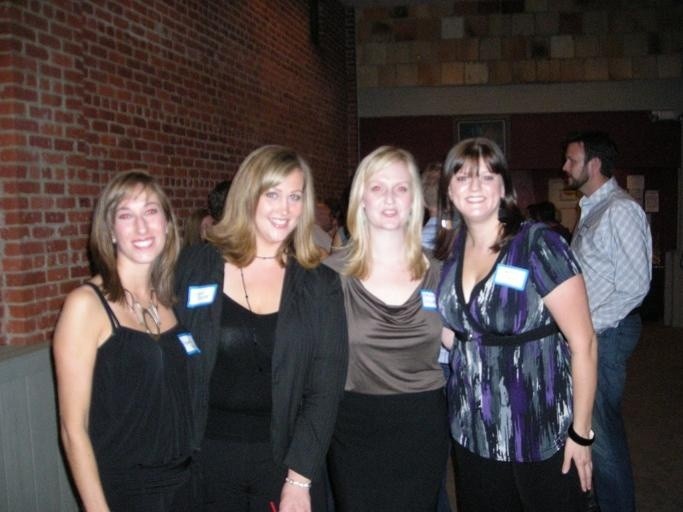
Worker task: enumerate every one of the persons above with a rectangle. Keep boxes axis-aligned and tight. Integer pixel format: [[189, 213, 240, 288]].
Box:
[[529, 200, 571, 244], [168, 143, 349, 512], [559, 131, 653, 510], [50, 167, 200, 512], [315, 196, 347, 252], [187, 181, 231, 247], [420, 164, 444, 252], [314, 142, 451, 510], [428, 137, 604, 512]]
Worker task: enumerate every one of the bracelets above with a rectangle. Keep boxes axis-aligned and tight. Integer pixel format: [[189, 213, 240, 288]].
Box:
[[567, 421, 596, 446], [282, 476, 311, 489]]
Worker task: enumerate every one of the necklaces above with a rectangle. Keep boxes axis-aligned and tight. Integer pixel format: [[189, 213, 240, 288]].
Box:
[[113, 281, 162, 343], [234, 249, 287, 377]]
